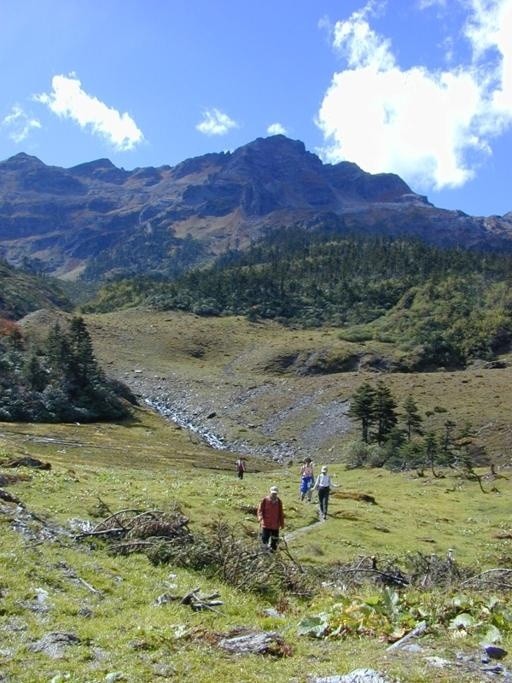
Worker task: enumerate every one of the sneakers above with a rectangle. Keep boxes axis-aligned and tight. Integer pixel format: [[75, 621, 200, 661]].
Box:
[[319, 513, 327, 519]]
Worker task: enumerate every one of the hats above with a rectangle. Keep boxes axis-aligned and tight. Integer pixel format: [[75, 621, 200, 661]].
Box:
[[321, 466, 328, 473], [304, 457, 311, 462], [271, 486, 278, 494]]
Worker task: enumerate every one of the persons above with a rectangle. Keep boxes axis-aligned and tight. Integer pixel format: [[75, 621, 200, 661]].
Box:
[[257, 484, 285, 553], [297, 456, 315, 503], [236, 456, 246, 479], [310, 465, 342, 519]]
